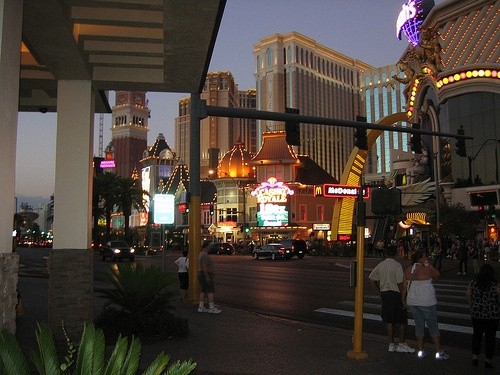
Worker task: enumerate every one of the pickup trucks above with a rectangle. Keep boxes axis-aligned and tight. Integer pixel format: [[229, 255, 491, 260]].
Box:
[[100, 241, 136, 263]]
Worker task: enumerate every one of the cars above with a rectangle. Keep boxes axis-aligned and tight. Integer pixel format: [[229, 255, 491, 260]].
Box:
[[207, 242, 234, 255], [251, 243, 291, 260]]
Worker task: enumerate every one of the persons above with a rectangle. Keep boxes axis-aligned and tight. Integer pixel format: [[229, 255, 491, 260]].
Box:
[[373, 229, 500, 276], [172, 251, 189, 298], [368, 246, 416, 353], [466, 263, 499, 369], [198, 242, 222, 313], [401, 251, 450, 360]]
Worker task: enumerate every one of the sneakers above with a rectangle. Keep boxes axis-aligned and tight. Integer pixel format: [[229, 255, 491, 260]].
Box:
[[416, 349, 427, 357], [396, 343, 416, 352], [388, 342, 396, 352], [198, 306, 208, 312], [207, 307, 223, 314], [434, 351, 451, 361]]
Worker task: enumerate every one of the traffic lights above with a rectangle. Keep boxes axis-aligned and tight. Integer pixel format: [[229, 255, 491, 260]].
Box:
[[487, 221, 498, 245], [283, 107, 301, 146], [455, 126, 467, 156], [409, 122, 422, 155], [353, 115, 368, 150]]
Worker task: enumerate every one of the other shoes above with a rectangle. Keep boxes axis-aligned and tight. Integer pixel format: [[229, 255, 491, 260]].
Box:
[[472, 358, 479, 364], [485, 359, 497, 368]]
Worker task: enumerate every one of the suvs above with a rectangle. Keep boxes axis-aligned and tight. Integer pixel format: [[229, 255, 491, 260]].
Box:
[[277, 239, 308, 259]]
